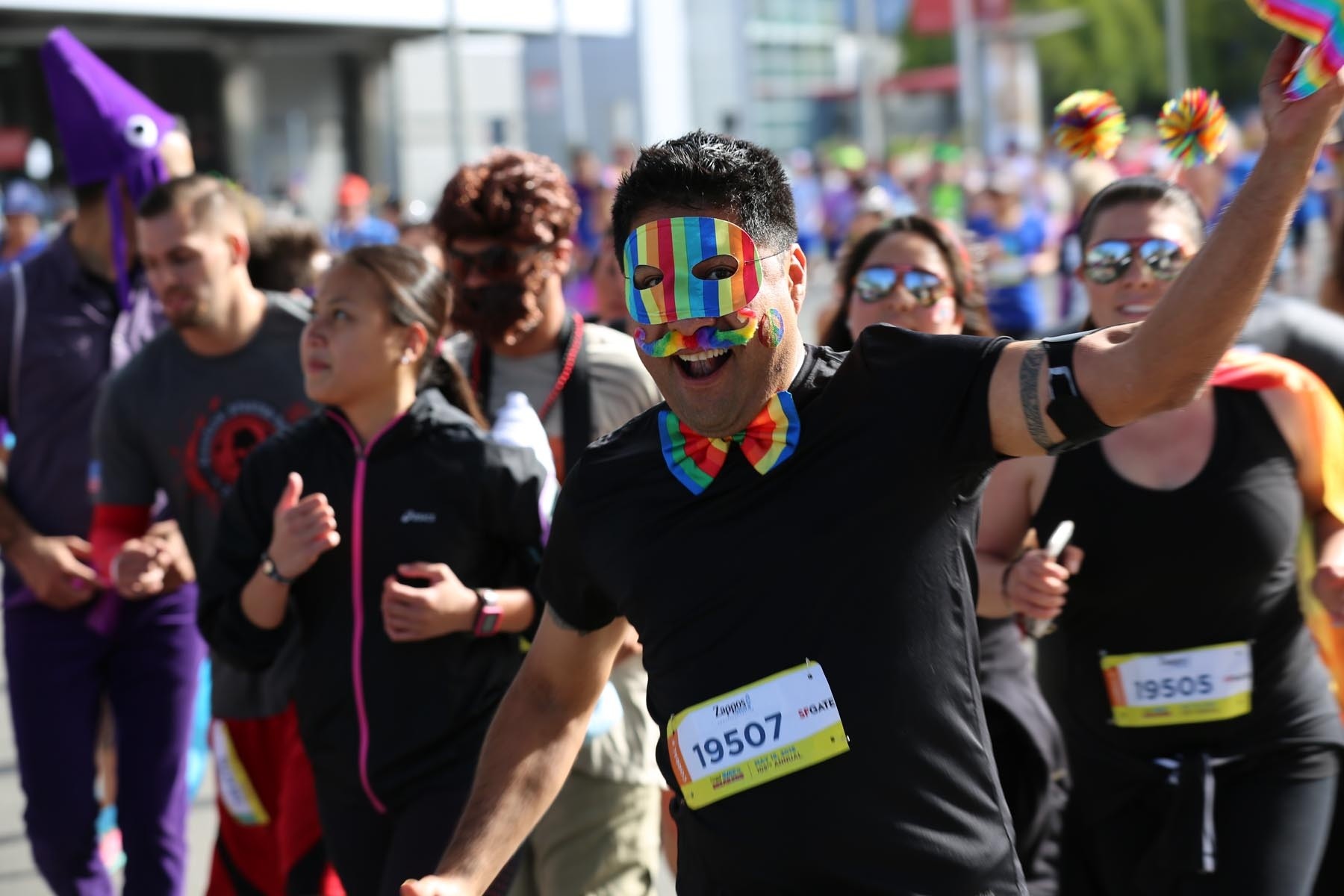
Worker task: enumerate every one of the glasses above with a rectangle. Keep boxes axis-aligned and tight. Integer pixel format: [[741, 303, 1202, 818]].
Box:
[[447, 243, 553, 282], [850, 263, 960, 305], [1081, 235, 1190, 284]]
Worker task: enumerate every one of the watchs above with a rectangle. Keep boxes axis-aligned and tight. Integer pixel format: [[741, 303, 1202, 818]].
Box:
[[473, 587, 504, 640], [259, 550, 294, 582]]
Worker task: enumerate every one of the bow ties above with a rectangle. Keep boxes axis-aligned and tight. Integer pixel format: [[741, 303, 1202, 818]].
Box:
[[659, 390, 800, 496]]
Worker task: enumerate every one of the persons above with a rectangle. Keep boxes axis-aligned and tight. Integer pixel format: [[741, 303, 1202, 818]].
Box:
[[1, 21, 1344, 895]]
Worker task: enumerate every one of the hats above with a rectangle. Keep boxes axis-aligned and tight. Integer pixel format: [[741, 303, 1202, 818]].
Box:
[[4, 180, 45, 214], [338, 176, 369, 206], [42, 27, 176, 312]]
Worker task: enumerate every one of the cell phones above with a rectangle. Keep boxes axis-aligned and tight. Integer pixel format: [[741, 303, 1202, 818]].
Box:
[[1027, 521, 1074, 637]]
